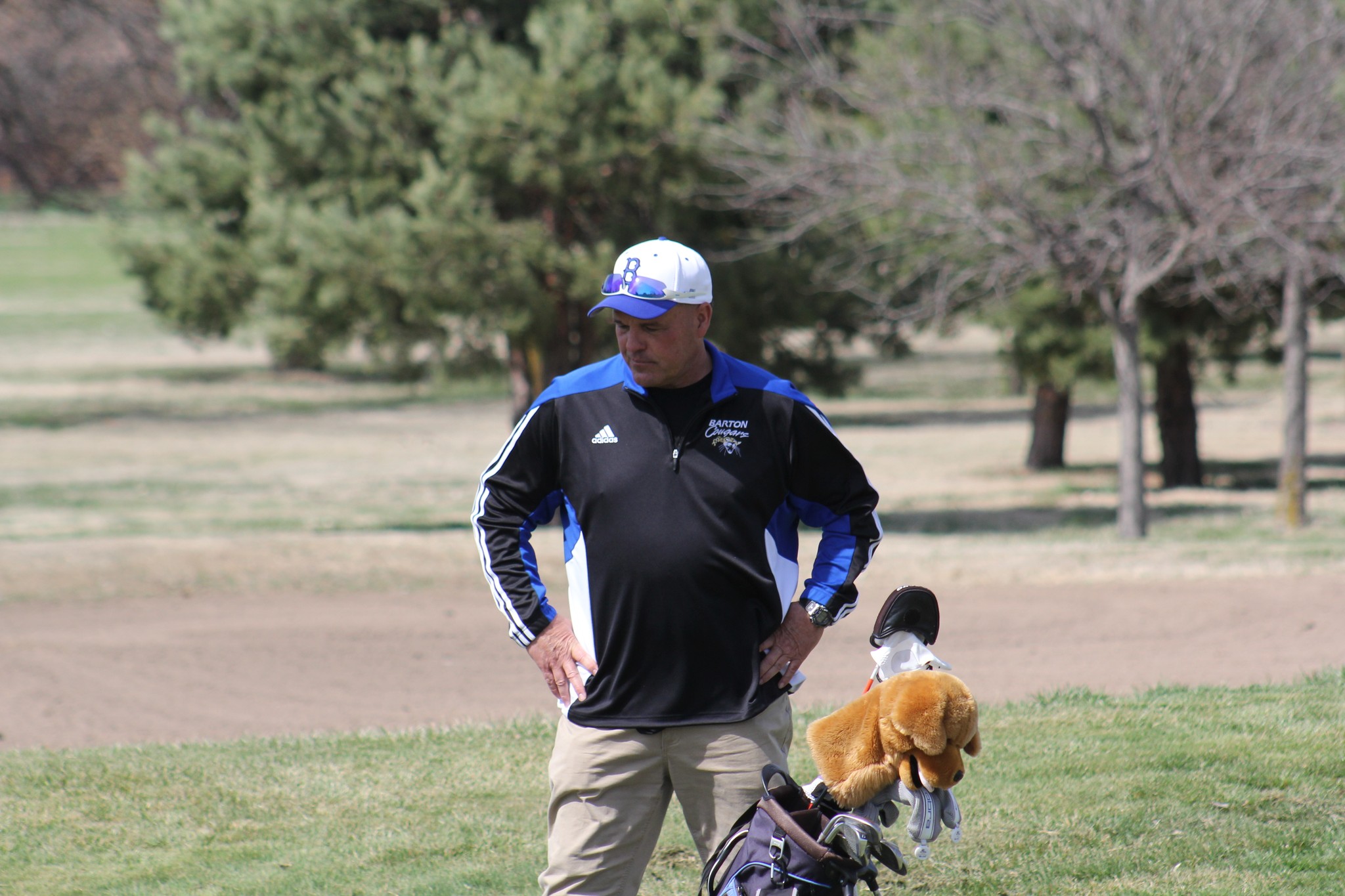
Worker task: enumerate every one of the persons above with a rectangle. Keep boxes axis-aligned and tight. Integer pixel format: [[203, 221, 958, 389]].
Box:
[[470, 236, 883, 896]]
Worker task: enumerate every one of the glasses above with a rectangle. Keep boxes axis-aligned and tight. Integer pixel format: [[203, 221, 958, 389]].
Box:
[[600, 272, 710, 300]]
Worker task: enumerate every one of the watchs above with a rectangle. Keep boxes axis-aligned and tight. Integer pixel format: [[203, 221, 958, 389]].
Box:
[[799, 598, 834, 628]]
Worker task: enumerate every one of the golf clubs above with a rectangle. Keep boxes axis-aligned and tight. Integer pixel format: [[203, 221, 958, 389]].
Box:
[[816, 810, 912, 878]]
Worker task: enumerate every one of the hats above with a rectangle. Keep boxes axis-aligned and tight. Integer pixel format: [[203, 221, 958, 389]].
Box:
[[589, 236, 714, 320]]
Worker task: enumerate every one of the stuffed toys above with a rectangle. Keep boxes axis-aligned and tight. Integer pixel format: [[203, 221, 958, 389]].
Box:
[[807, 672, 984, 807]]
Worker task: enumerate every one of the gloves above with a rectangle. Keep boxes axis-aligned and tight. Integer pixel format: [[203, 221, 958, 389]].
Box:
[[872, 631, 952, 683]]
